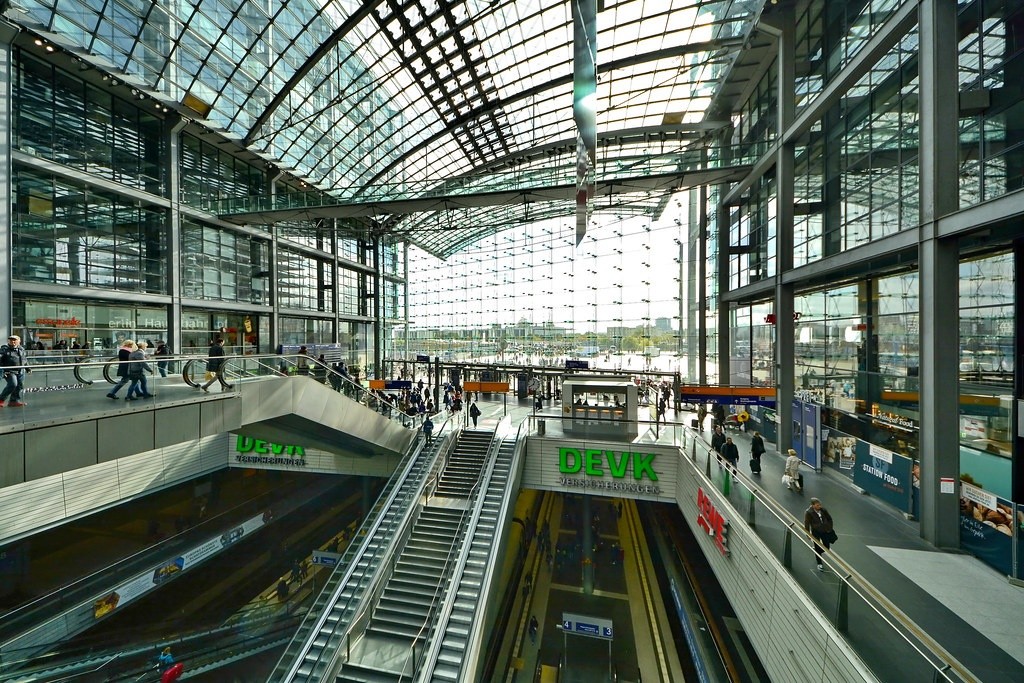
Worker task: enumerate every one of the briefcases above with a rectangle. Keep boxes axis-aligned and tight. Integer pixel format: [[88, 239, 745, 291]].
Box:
[[161, 663, 183, 683]]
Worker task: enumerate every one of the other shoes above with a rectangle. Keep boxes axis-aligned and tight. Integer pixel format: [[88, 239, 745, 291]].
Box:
[[797, 487, 801, 491], [787, 486, 791, 489], [719, 465, 722, 469], [733, 478, 739, 483]]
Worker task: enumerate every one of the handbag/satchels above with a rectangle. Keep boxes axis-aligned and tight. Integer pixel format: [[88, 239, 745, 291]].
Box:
[[782, 475, 792, 488], [829, 530, 838, 544], [205, 372, 215, 381], [206, 363, 225, 372]]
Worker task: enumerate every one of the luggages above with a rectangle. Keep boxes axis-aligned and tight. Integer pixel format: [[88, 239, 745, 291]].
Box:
[[711, 418, 717, 430], [749, 452, 762, 473], [692, 419, 699, 427], [798, 473, 803, 488]]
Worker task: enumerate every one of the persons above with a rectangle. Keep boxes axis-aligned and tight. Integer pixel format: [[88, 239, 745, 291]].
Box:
[[524, 511, 620, 645], [26, 339, 44, 363], [785, 450, 801, 491], [148, 479, 222, 539], [202, 338, 226, 391], [277, 344, 473, 446], [113, 332, 126, 348], [470, 403, 479, 427], [751, 431, 765, 463], [159, 647, 174, 673], [107, 340, 172, 400], [52, 340, 89, 364], [647, 379, 670, 425], [697, 403, 739, 482], [828, 439, 853, 462], [829, 380, 851, 397], [0, 335, 31, 408], [259, 525, 350, 612], [805, 498, 833, 571], [474, 349, 661, 406]]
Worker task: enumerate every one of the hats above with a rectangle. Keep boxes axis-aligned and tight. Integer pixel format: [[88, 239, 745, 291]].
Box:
[[8, 336, 19, 339]]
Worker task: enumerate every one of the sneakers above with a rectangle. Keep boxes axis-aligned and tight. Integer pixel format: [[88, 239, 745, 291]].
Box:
[[144, 394, 156, 399], [125, 395, 138, 401], [8, 402, 27, 407], [107, 393, 120, 399], [0, 400, 4, 407], [137, 393, 144, 397], [817, 564, 824, 572], [202, 386, 210, 393]]
